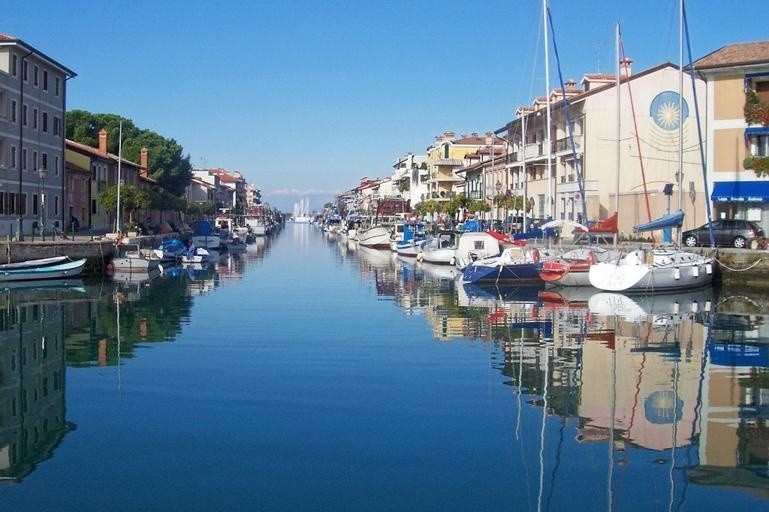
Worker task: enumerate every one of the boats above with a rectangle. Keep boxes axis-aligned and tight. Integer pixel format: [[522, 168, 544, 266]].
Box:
[[109, 208, 284, 273], [112, 267, 160, 284], [0, 281, 88, 294], [0, 255, 88, 281], [180, 263, 207, 275]]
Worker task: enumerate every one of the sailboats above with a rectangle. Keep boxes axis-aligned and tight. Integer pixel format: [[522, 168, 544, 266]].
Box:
[[289, 200, 309, 222]]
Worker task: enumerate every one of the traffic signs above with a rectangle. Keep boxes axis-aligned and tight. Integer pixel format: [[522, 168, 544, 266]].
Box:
[[38, 165, 48, 241]]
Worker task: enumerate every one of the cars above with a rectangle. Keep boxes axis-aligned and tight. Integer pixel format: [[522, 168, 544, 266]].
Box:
[[682, 219, 764, 248], [693, 310, 762, 332]]
